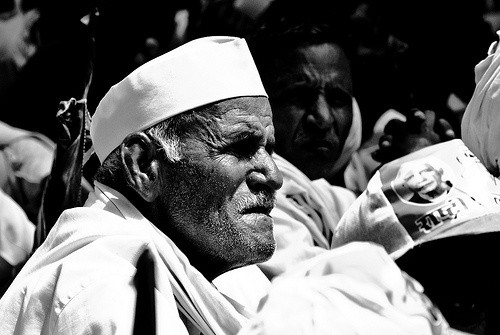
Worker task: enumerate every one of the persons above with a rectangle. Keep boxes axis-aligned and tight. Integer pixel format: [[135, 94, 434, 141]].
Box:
[[401, 162, 453, 205], [0, 0, 499, 335]]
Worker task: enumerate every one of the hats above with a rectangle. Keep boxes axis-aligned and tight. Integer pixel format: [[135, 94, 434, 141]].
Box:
[[88, 36, 269, 166], [329, 138, 499, 260]]
